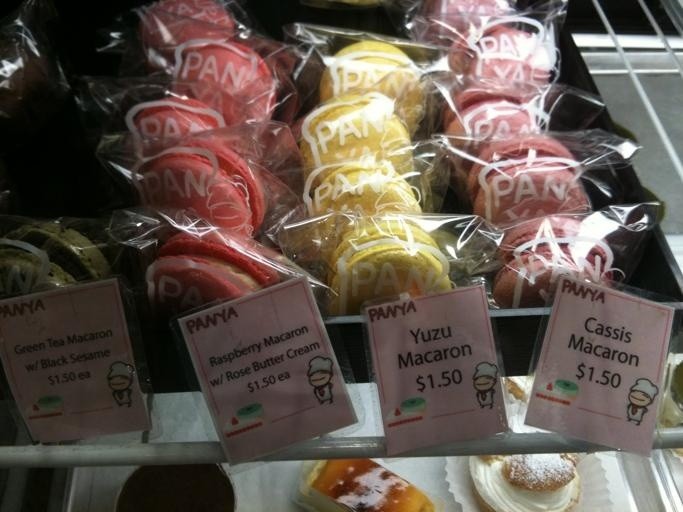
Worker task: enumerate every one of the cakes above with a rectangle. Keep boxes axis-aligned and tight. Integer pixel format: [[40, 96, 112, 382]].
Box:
[[225, 416, 262, 437], [297, 456, 438, 511], [479, 376, 578, 492], [657, 357, 683, 462], [387, 407, 422, 427], [536, 383, 571, 407], [22, 404, 66, 420]]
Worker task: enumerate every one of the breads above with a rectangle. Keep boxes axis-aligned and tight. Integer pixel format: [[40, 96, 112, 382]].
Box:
[[1, 207, 109, 302]]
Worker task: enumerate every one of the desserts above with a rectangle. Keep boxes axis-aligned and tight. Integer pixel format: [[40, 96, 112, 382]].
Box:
[[133, 0, 279, 303], [424, 0, 614, 308], [296, 37, 455, 319]]
